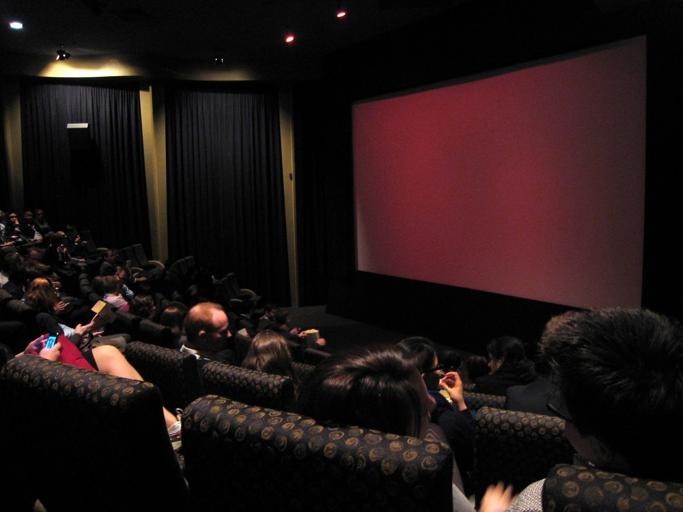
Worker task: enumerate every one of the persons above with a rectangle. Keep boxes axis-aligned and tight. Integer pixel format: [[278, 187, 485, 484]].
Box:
[[0, 203, 546, 512], [504, 304, 681, 510]]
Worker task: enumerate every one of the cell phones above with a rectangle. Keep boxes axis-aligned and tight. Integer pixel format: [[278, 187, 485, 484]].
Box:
[[79, 310, 97, 326], [45, 332, 58, 349]]
